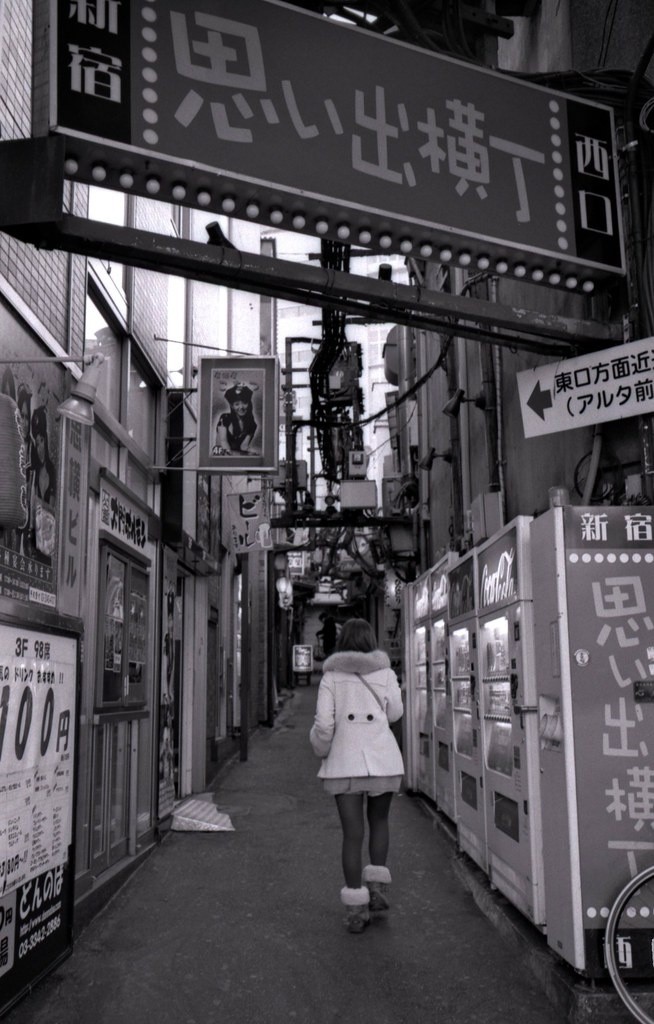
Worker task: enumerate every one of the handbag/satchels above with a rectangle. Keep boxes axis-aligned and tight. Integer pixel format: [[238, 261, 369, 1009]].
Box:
[[313, 636, 326, 662]]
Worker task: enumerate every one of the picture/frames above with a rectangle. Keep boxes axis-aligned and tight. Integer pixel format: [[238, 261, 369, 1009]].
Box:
[[198, 354, 280, 475]]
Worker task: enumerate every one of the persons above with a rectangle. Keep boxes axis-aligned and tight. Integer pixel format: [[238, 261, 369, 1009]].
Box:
[[314, 613, 338, 657], [309, 618, 406, 935], [215, 385, 258, 457], [161, 588, 173, 782], [2, 367, 57, 508]]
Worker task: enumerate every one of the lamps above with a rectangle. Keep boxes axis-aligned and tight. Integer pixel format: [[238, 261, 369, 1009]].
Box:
[[369, 263, 405, 312], [206, 222, 236, 249], [0, 351, 106, 425], [442, 389, 487, 418], [418, 448, 453, 472]]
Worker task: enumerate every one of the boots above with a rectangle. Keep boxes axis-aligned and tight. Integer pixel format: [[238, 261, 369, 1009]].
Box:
[[341, 885, 371, 935], [362, 864, 392, 912]]
